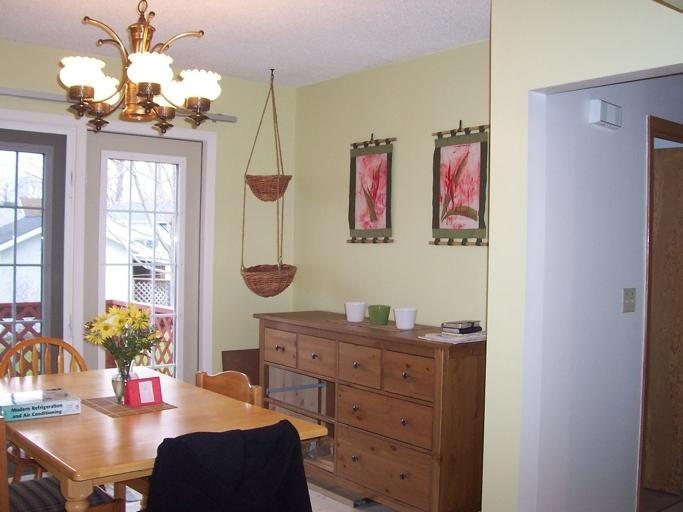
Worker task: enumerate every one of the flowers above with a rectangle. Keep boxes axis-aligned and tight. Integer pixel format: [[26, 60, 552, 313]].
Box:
[[81, 304, 163, 383]]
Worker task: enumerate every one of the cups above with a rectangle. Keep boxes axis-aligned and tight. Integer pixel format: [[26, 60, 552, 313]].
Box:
[[344, 301, 365, 323], [367, 304, 391, 324], [110, 374, 124, 404], [393, 308, 417, 330]]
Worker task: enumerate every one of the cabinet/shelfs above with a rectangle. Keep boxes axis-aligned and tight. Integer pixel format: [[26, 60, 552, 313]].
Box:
[[251, 309, 483, 511]]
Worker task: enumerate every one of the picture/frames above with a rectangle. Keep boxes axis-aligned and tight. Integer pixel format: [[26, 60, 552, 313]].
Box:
[[347, 135, 396, 246], [425, 124, 489, 248]]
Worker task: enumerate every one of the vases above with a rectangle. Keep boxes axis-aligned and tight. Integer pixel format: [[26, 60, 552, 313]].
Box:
[[110, 358, 138, 406]]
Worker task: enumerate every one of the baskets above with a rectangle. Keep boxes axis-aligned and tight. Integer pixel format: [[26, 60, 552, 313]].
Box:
[[241, 263, 298, 299], [245, 173, 292, 204]]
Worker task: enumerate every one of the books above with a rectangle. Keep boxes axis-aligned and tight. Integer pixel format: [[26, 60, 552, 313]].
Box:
[[442, 320, 482, 333], [0, 390, 81, 423]]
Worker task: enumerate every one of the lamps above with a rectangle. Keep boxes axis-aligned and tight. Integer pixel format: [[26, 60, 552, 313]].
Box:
[[57, 1, 238, 134]]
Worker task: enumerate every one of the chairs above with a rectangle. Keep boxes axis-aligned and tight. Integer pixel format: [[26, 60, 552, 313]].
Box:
[[0, 415, 126, 511], [111, 369, 266, 512], [0, 337, 87, 485], [144, 420, 304, 512]]
